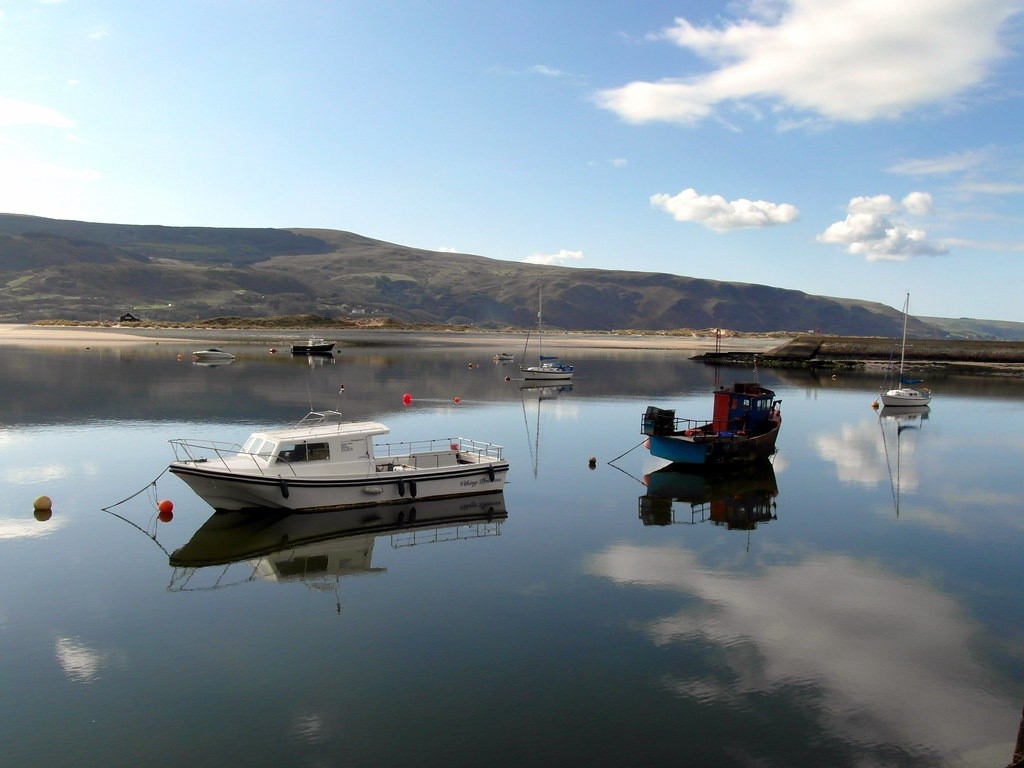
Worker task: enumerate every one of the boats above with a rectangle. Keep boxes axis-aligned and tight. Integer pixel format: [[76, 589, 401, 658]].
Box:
[[166, 407, 509, 512], [638, 379, 781, 466], [193, 348, 236, 359], [639, 445, 778, 550], [291, 351, 335, 367], [494, 361, 514, 366], [291, 335, 335, 352], [494, 353, 514, 360], [191, 359, 235, 367], [167, 493, 508, 614]]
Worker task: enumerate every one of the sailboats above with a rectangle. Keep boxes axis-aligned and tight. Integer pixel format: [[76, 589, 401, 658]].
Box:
[[520, 280, 574, 380], [880, 290, 933, 407], [878, 406, 932, 517], [518, 380, 575, 478]]
